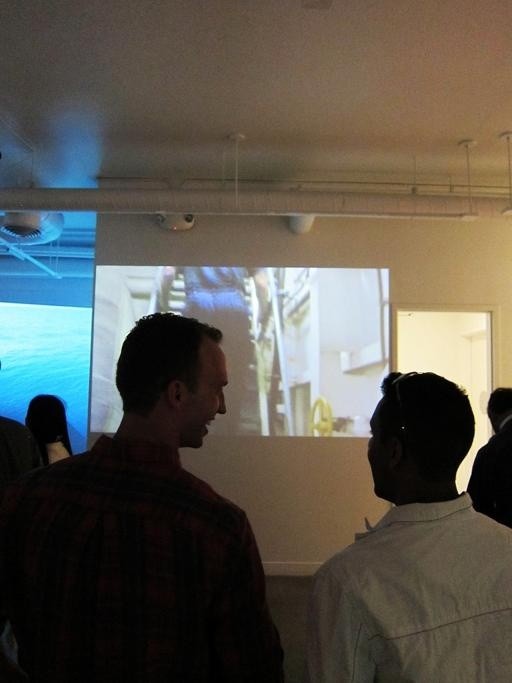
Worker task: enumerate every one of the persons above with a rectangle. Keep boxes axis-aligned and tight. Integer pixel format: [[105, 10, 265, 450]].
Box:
[[0, 310, 292, 681], [156, 267, 273, 428], [23, 394, 76, 471], [309, 361, 512, 681], [459, 384, 510, 531]]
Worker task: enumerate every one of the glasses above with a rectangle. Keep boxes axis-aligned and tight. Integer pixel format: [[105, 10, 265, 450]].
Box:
[[391, 371, 420, 442]]
